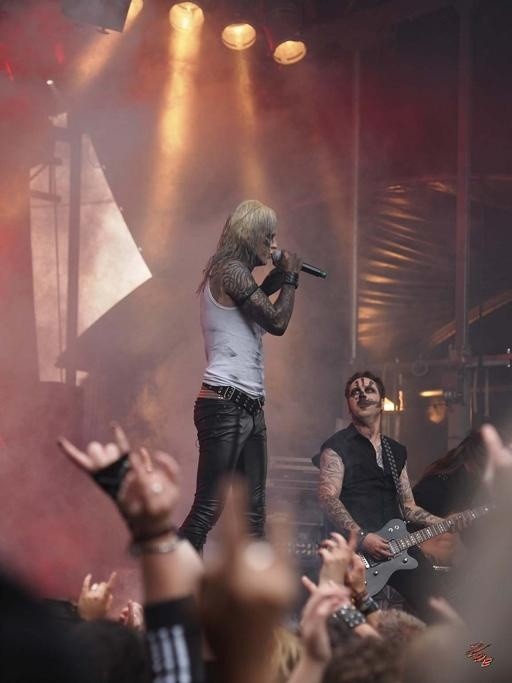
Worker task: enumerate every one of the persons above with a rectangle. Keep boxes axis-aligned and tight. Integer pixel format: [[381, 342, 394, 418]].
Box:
[[307, 371, 477, 621], [176, 198, 302, 558]]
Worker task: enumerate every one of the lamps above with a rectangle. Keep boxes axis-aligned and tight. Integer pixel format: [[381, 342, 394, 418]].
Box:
[[162, 0, 309, 66]]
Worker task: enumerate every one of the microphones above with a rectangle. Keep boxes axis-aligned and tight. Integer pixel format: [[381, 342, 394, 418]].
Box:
[[271, 250, 327, 278]]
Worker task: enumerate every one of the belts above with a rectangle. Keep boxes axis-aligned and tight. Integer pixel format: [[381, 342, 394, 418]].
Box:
[[198, 382, 264, 416]]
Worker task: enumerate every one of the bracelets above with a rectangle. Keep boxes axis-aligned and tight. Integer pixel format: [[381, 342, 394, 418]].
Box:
[[279, 270, 300, 289]]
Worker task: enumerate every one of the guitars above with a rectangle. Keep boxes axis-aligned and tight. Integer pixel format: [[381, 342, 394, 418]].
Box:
[[357, 499, 500, 597]]
